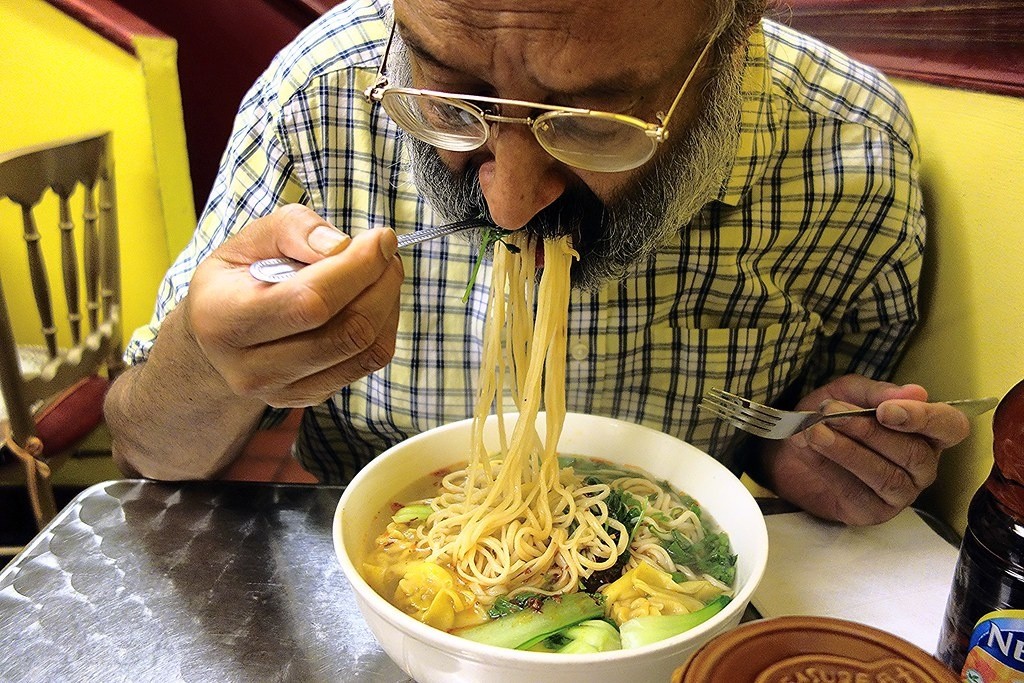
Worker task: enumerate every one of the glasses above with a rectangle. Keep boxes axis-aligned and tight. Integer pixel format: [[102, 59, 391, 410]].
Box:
[[366, 12, 718, 172]]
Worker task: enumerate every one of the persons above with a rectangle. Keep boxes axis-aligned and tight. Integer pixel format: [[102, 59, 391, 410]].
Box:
[[95, 2, 972, 529]]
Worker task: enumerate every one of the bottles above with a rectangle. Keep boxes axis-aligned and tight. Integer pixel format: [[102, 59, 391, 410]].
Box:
[[926, 373, 1024, 682]]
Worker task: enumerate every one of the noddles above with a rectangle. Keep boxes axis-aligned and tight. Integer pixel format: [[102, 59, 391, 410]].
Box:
[[361, 234, 736, 654]]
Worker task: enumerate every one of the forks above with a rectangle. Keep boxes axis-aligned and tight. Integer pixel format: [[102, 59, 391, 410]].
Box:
[[695, 386, 1000, 439]]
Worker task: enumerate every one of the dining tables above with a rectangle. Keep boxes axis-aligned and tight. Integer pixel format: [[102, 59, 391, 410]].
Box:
[[0, 478, 966, 683]]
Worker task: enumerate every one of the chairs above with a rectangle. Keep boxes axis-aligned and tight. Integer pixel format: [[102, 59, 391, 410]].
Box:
[[1, 131, 132, 547]]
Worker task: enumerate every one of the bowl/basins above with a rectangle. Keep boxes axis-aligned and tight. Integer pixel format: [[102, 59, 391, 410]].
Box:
[[331, 411, 769, 683]]
